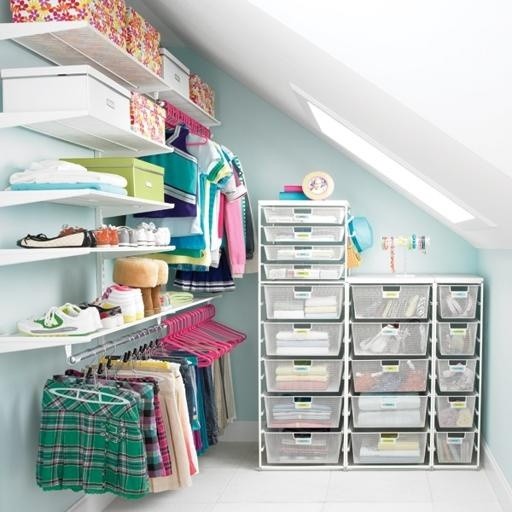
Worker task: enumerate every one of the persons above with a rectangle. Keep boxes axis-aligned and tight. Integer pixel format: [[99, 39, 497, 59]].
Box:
[[311, 179, 324, 193]]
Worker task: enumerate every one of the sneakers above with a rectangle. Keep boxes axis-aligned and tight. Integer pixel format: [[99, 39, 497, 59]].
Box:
[[17, 283, 145, 336], [95, 221, 171, 247]]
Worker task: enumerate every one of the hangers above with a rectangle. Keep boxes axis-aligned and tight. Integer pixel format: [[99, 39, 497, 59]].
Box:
[[48, 305, 247, 406], [163, 101, 212, 147]]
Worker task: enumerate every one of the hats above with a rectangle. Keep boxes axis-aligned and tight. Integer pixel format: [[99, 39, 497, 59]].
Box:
[[346, 213, 374, 253]]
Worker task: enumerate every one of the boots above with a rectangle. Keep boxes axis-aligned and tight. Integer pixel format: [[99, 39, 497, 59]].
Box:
[[147, 258, 169, 313], [113, 258, 159, 317]]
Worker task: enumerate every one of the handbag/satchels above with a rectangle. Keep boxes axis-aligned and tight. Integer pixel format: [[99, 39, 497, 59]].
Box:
[[347, 244, 361, 268]]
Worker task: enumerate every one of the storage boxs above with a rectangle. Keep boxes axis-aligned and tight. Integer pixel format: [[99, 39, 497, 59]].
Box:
[[189, 74, 216, 121], [61, 157, 166, 202], [9, 0, 127, 48], [0, 64, 131, 129], [160, 48, 190, 97], [131, 94, 166, 143], [127, 7, 162, 74]]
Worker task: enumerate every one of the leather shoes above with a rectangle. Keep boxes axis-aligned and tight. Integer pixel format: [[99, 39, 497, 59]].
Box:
[[20, 231, 91, 248], [36, 230, 97, 247]]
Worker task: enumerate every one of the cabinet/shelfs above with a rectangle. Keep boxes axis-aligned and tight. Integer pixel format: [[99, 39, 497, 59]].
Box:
[[0, 22, 222, 355]]
